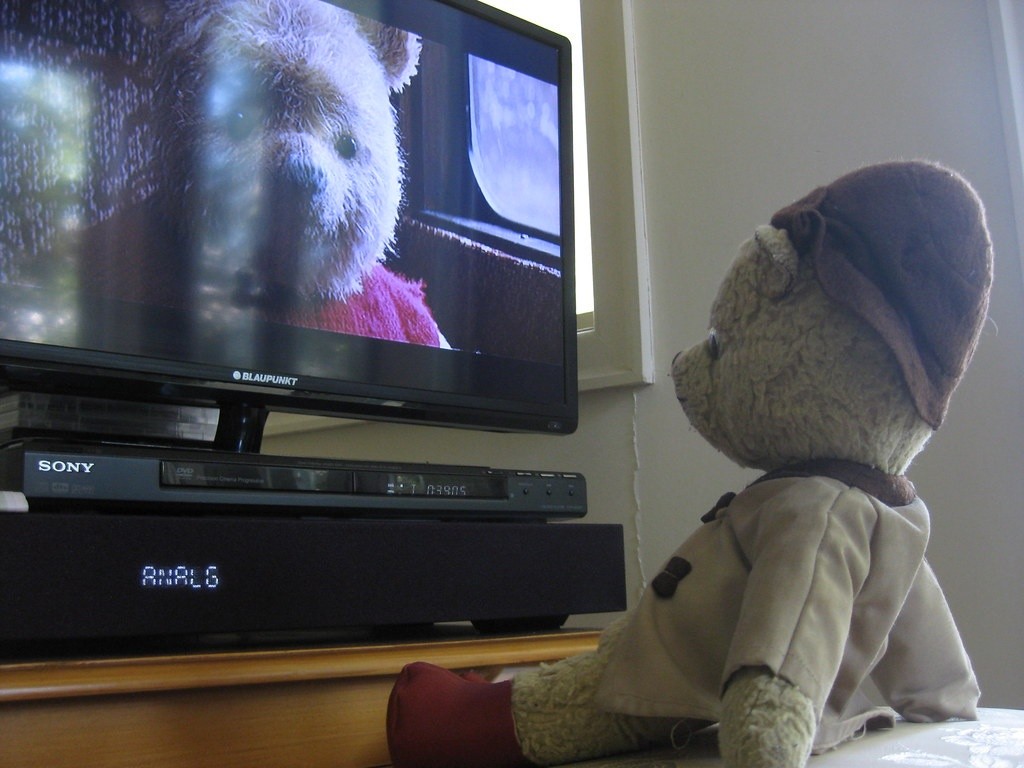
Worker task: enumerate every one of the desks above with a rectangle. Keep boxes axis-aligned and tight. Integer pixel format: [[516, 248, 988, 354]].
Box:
[[0, 628, 627, 768]]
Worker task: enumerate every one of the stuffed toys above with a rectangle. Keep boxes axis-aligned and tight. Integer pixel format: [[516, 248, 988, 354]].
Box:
[[385, 160, 994, 768], [77, 0, 451, 350]]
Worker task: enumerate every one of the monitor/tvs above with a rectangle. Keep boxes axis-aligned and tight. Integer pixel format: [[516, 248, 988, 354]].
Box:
[[0, 0, 578, 455]]
